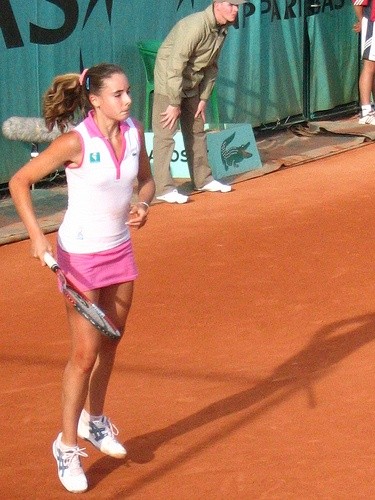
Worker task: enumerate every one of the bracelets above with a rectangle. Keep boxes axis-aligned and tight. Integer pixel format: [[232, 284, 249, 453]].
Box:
[[138, 202, 150, 215]]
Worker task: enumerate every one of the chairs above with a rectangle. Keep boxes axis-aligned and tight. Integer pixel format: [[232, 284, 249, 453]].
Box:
[[136, 39, 220, 132]]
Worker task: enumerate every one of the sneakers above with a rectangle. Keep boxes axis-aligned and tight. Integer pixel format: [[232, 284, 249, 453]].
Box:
[[358, 110, 375, 126], [192, 179, 233, 193], [52, 432, 88, 494], [76, 414, 128, 459], [156, 188, 190, 205]]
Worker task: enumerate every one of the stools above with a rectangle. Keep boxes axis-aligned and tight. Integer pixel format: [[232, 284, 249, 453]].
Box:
[[2, 116, 64, 190]]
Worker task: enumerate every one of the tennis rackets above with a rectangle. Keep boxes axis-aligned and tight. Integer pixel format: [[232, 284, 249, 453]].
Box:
[[43, 251, 121, 339]]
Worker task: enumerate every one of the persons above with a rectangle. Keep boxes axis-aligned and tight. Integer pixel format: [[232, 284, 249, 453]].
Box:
[[352, 0, 375, 126], [9, 65, 157, 495], [152, 0, 249, 203]]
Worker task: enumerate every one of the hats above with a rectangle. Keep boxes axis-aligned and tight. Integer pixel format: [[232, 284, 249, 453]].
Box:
[[211, 0, 248, 5]]
